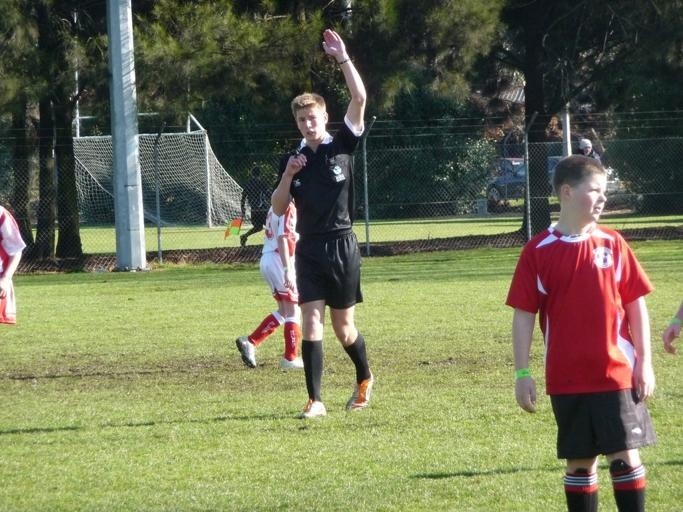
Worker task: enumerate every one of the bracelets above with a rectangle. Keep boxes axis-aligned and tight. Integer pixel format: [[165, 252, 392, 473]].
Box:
[[668, 318, 683, 327], [513, 367, 531, 381], [336, 56, 350, 65]]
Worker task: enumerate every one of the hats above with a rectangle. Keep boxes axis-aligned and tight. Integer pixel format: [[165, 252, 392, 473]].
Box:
[[579, 139, 592, 150]]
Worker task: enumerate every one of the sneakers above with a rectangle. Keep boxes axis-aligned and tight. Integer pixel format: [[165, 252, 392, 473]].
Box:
[[240, 234, 248, 247], [298, 399, 326, 418], [280, 356, 305, 368], [236, 337, 257, 368], [346, 369, 373, 411]]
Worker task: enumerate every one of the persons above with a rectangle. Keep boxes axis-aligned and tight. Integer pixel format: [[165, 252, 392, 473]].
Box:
[[578, 137, 602, 167], [662, 299, 683, 356], [271, 29, 376, 419], [240, 167, 272, 247], [504, 153, 655, 512], [235, 196, 307, 369], [0, 204, 28, 326]]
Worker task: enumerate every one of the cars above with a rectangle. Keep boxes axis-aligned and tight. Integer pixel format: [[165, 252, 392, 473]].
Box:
[[485, 155, 620, 203]]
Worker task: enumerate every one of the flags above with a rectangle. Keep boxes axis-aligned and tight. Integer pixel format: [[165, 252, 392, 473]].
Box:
[[222, 215, 243, 243]]
